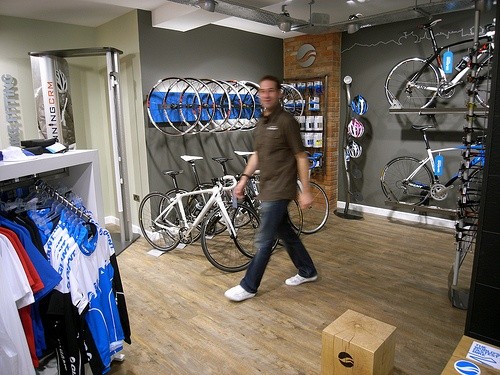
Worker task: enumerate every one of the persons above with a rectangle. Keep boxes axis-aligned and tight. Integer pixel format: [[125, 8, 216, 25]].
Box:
[[225, 75, 318, 301]]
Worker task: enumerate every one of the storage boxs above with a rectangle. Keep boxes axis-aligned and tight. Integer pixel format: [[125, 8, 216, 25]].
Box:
[[441, 335, 500, 375]]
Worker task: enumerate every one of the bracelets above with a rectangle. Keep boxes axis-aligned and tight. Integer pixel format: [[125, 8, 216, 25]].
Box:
[[241, 174, 251, 179]]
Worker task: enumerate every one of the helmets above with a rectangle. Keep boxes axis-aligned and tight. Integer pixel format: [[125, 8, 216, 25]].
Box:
[[346, 141, 362, 158], [348, 120, 365, 138], [350, 95, 368, 116]]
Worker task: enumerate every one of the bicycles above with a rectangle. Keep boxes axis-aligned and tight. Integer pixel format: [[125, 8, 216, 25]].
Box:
[[379, 124, 483, 214], [382, 18, 496, 109], [137, 150, 330, 273]]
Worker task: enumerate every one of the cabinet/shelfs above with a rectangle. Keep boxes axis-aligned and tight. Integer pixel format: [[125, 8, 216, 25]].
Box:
[[464, 5, 500, 349], [383, 107, 489, 217]]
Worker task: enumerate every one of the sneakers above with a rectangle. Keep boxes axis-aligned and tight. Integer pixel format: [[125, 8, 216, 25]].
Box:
[[285, 273, 317, 285], [224, 285, 256, 302]]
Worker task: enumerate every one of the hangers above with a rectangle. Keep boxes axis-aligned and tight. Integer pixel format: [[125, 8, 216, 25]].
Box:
[[26, 176, 94, 230]]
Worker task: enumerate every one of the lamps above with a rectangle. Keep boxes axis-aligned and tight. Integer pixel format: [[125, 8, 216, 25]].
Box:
[[277, 5, 291, 33], [347, 14, 359, 34], [196, 0, 218, 12]]
